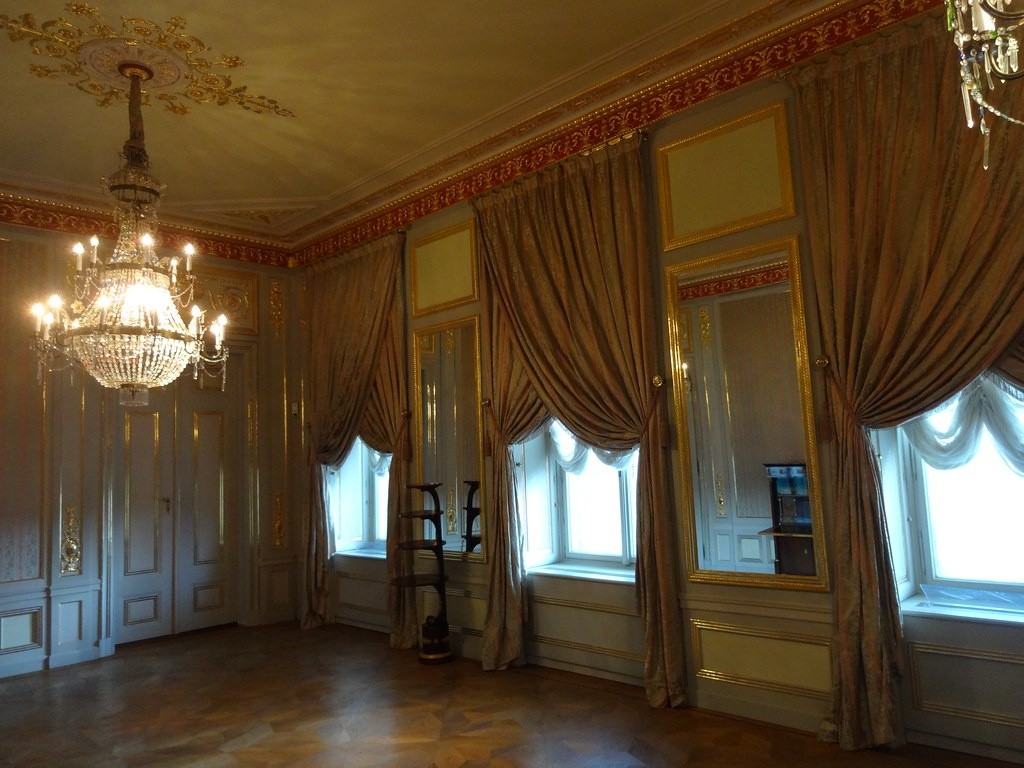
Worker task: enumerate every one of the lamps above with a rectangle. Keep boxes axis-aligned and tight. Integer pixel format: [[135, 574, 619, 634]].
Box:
[[31, 57, 230, 407], [943, 0, 1024, 170]]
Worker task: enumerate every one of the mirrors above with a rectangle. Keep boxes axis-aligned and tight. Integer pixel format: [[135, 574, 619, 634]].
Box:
[[411, 314, 488, 564], [665, 234, 831, 592]]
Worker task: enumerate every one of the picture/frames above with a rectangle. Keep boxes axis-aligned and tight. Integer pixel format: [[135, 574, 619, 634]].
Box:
[[655, 98, 797, 254], [409, 215, 480, 318]]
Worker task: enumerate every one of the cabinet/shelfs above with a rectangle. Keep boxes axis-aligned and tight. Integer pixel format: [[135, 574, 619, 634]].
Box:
[[389, 482, 454, 666], [463, 480, 482, 552], [759, 461, 817, 577]]
